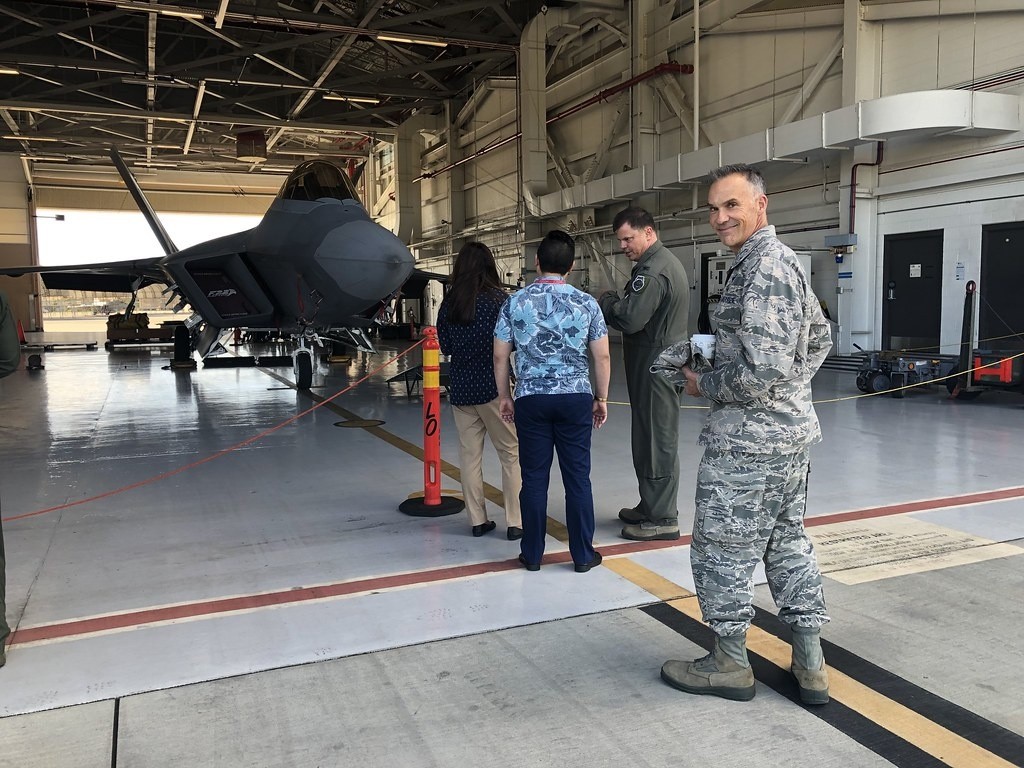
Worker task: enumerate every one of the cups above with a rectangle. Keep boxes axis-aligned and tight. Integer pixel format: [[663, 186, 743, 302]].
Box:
[[690, 333, 716, 373]]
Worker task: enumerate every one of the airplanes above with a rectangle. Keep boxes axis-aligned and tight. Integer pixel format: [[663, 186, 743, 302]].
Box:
[[1, 145, 527, 393]]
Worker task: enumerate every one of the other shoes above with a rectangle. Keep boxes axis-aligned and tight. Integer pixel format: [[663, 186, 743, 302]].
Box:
[[618, 508, 679, 524], [507, 527, 522, 540], [575, 551, 603, 573], [621, 520, 680, 540], [519, 553, 540, 571], [472, 520, 496, 537]]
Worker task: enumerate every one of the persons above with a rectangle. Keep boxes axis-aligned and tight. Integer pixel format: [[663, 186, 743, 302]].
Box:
[[436, 243, 523, 542], [598, 206, 689, 542], [659, 163, 835, 704], [492, 230, 611, 571]]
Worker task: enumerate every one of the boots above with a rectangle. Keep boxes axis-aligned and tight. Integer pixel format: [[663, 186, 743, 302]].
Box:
[[791, 632, 829, 707], [661, 636, 756, 702]]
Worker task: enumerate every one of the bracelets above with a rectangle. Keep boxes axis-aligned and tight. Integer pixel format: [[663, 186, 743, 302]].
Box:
[[595, 396, 607, 402]]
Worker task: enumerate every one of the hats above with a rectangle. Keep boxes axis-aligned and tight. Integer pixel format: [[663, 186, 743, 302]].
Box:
[[649, 340, 714, 387]]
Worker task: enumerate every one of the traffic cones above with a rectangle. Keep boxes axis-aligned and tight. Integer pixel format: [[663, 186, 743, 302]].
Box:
[[16, 319, 27, 346]]
